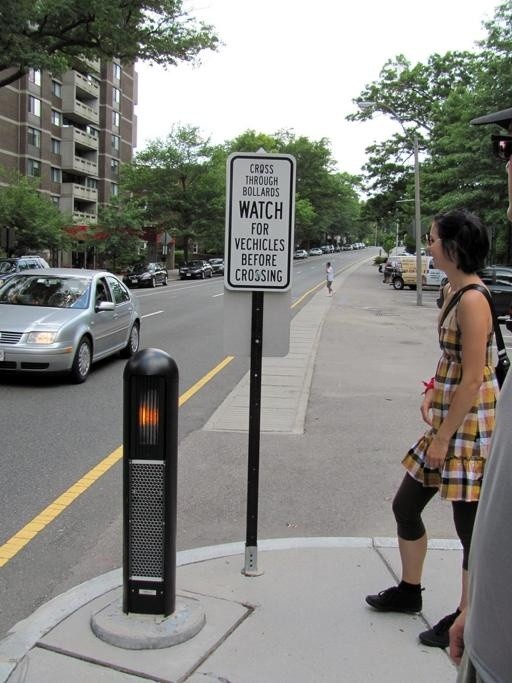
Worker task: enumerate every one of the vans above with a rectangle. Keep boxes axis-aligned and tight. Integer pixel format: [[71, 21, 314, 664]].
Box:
[[379, 247, 512, 330]]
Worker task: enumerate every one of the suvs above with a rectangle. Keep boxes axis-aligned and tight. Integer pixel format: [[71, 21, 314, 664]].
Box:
[[0, 253, 55, 290]]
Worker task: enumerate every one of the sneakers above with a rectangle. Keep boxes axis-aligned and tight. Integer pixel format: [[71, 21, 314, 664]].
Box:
[[362, 583, 425, 614], [416, 608, 462, 650]]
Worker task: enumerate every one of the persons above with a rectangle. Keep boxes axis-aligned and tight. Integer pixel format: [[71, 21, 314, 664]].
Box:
[[449, 109, 512, 683], [364, 209, 502, 646], [326, 262, 336, 297]]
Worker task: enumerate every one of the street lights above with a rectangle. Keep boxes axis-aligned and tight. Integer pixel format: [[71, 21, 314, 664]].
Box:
[[395, 216, 401, 246], [355, 99, 424, 306]]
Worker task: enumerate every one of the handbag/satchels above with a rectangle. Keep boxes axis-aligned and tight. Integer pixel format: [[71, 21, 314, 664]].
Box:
[[494, 353, 510, 393]]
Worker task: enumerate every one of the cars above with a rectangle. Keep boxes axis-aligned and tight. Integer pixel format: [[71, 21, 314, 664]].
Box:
[[0, 266, 142, 385], [309, 241, 366, 256], [293, 248, 308, 258], [122, 256, 224, 288]]
